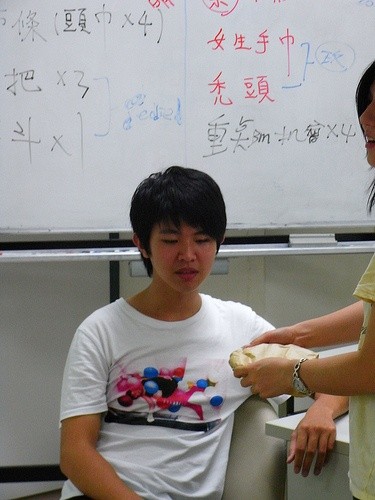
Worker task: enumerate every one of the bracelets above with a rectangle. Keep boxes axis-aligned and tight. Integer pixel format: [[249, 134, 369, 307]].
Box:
[[292, 358, 313, 396]]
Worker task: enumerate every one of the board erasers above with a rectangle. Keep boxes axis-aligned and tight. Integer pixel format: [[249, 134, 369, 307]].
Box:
[[288, 237, 337, 247]]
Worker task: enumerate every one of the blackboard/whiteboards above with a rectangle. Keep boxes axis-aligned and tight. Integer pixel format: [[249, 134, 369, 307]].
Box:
[[0, 0, 375, 262]]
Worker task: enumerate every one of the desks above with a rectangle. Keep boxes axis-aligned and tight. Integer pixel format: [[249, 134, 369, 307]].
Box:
[[265, 411, 353, 500]]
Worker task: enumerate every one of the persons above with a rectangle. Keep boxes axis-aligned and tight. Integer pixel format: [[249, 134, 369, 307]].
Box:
[[231, 59, 375, 500], [56, 165, 350, 500]]
